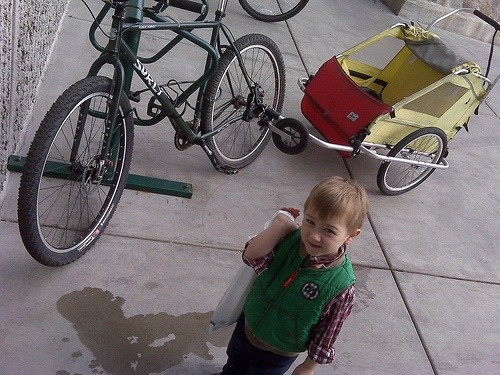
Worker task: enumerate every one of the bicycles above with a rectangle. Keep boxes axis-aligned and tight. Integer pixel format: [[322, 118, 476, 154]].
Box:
[[17, 1, 288, 267], [240, 0, 309, 21]]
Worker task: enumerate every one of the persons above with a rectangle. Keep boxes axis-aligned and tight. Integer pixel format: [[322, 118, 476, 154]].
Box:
[[220, 174, 370, 375]]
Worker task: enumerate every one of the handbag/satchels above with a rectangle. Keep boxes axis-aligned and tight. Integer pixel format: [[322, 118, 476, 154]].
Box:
[[208, 209, 295, 331]]
